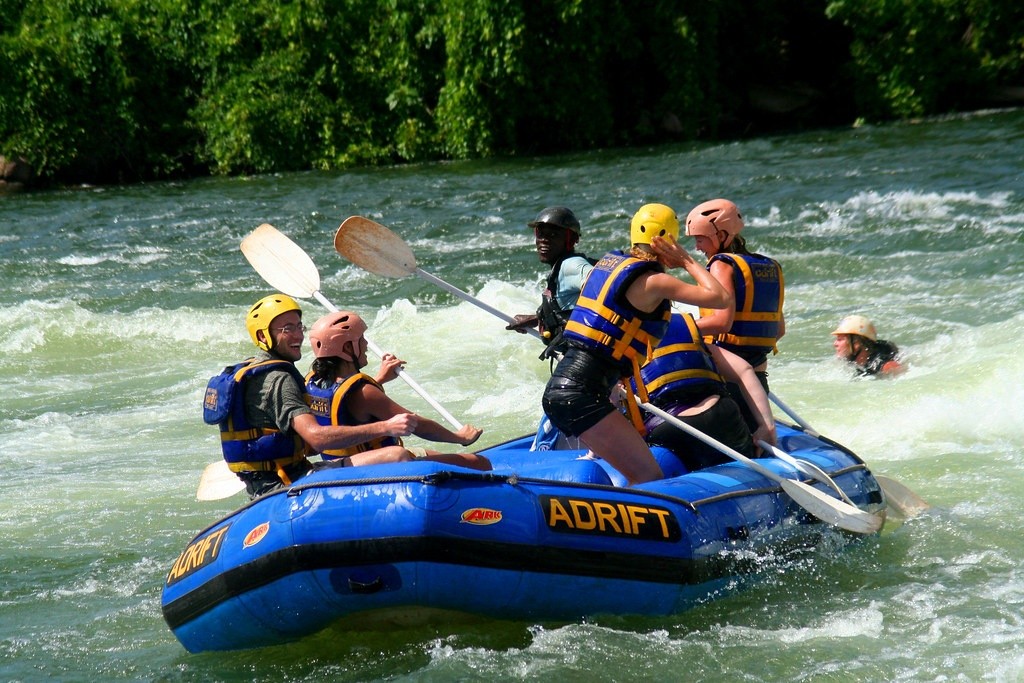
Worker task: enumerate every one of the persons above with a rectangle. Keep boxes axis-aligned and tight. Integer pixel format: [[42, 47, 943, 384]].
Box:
[[505, 206, 599, 449], [830, 315, 900, 377], [219, 294, 419, 503], [305, 310, 493, 472], [617, 199, 786, 473], [541, 203, 730, 486]]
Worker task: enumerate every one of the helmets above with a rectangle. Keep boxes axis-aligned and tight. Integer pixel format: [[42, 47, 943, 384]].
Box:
[[312, 314, 367, 362], [685, 199, 744, 251], [831, 315, 877, 343], [527, 206, 582, 237], [246, 293, 302, 352], [630, 203, 679, 248]]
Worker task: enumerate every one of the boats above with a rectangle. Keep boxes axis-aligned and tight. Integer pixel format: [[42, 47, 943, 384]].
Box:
[[162, 418, 885, 654]]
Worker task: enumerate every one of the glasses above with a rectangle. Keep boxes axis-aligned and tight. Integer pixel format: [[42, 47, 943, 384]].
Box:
[[272, 325, 306, 333]]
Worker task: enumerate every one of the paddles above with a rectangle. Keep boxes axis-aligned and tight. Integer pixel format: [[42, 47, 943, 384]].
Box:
[[238, 222, 465, 431], [196, 459, 248, 502], [332, 214, 543, 341], [768, 389, 929, 518], [537, 330, 885, 535]]
